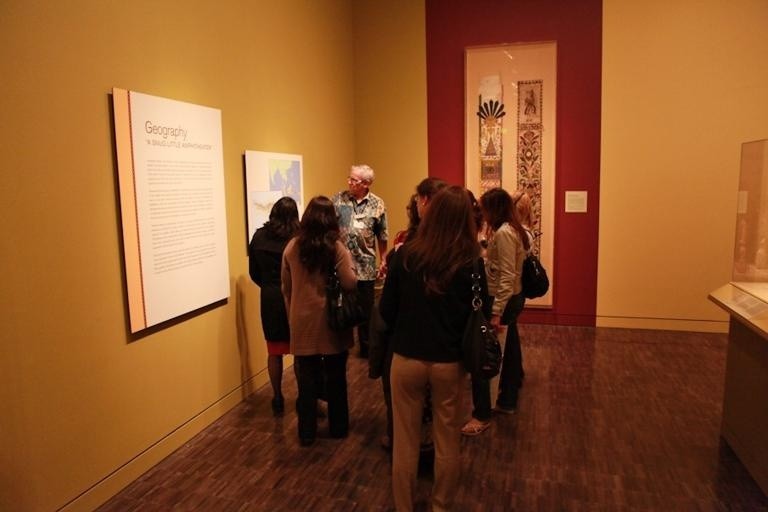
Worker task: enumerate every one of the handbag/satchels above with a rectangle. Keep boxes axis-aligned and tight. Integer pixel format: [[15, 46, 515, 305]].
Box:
[[522, 255, 549, 299], [473, 319, 502, 379], [327, 285, 371, 329]]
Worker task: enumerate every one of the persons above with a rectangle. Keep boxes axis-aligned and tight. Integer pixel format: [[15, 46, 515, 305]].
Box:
[[329, 164, 388, 360], [249, 196, 353, 445], [383, 176, 533, 510]]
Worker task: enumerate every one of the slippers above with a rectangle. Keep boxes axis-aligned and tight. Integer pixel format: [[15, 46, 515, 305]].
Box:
[[462, 420, 490, 435]]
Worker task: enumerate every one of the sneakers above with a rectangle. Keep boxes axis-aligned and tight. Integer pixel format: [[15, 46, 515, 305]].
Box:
[[272, 395, 284, 411]]
